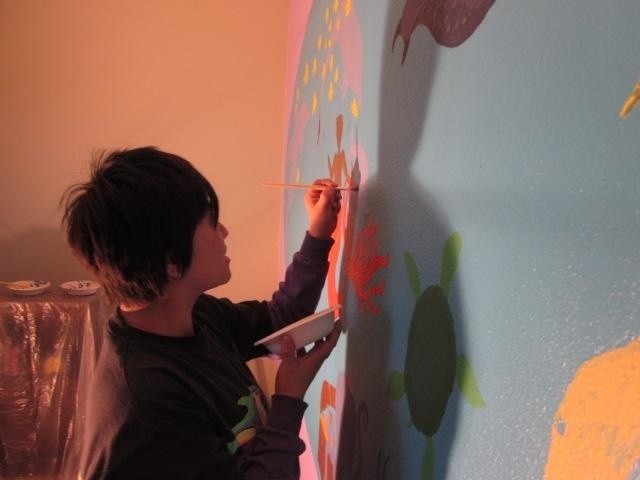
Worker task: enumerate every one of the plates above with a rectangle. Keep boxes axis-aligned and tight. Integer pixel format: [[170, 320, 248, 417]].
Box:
[[251, 301, 345, 356], [3, 277, 102, 297]]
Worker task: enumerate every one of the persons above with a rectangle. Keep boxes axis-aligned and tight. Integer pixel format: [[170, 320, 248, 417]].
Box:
[[61, 146, 343, 479]]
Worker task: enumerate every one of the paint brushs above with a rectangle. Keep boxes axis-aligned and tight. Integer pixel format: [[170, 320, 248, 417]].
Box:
[[264, 183, 359, 192]]
